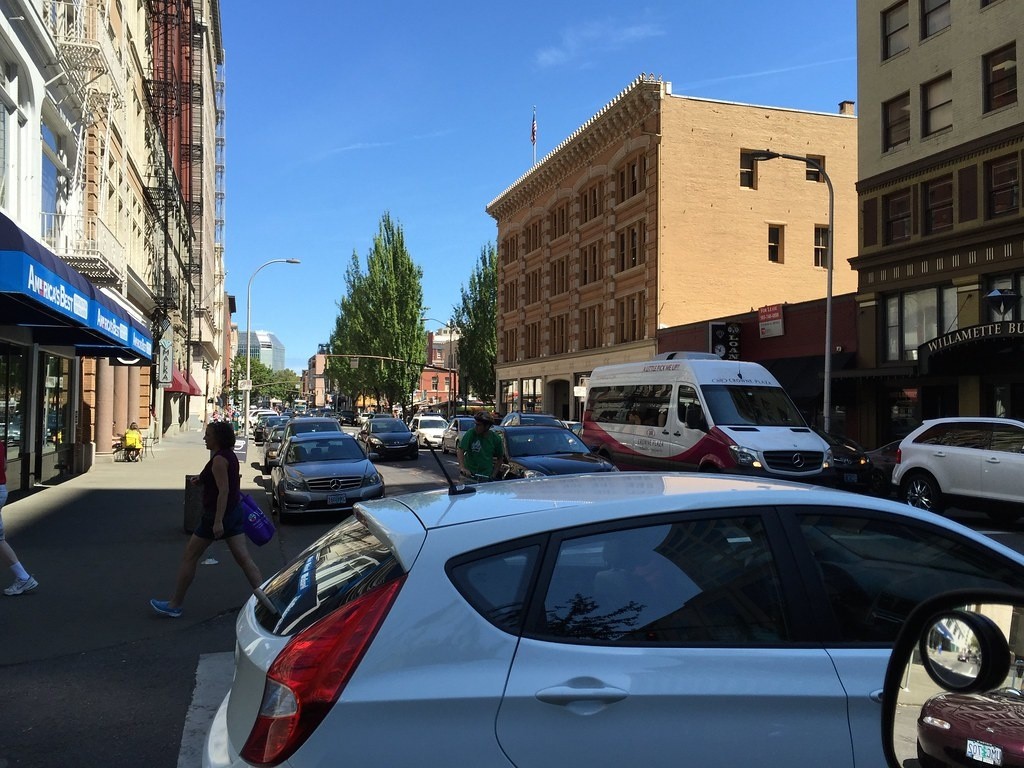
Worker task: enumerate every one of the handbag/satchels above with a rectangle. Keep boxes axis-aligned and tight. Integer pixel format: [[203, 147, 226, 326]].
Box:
[[239, 492, 276, 546]]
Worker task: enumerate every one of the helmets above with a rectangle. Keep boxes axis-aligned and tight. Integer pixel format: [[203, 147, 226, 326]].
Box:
[[474, 411, 495, 424]]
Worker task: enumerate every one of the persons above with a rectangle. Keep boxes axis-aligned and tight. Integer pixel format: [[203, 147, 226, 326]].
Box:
[[0, 437, 38, 596], [149, 421, 262, 619], [456, 412, 504, 484], [124, 422, 142, 462], [211, 404, 245, 426]]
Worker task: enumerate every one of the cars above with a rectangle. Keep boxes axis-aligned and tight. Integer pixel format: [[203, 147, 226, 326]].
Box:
[[498, 411, 565, 446], [238, 403, 392, 473], [270, 414, 355, 462], [958, 653, 978, 663], [269, 431, 387, 526], [355, 418, 420, 464], [489, 425, 621, 482], [0, 407, 67, 445], [914, 684, 1024, 768], [441, 418, 476, 456], [408, 414, 453, 449], [817, 430, 885, 499], [560, 420, 583, 441], [860, 436, 906, 501], [200, 468, 1024, 768]]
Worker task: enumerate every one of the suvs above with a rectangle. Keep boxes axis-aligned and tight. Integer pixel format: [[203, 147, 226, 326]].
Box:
[[891, 416, 1024, 534]]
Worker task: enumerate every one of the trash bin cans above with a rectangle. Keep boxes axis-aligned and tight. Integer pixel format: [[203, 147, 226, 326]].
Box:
[[230, 421, 238, 431], [184, 473, 243, 536]]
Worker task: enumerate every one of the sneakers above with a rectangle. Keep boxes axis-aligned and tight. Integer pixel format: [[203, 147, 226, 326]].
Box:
[[150, 599, 184, 617], [4, 576, 39, 595]]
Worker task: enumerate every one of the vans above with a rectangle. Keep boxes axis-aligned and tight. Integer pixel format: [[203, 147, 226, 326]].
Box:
[[580, 351, 835, 487]]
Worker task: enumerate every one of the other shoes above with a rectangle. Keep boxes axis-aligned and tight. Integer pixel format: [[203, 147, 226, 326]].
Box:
[[134, 457, 138, 462], [127, 456, 132, 461]]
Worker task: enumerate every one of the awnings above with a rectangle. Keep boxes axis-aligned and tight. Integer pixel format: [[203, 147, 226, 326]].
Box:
[[917, 320, 1024, 374], [165, 365, 202, 396], [80, 272, 129, 346], [0, 214, 89, 331], [129, 316, 152, 361]]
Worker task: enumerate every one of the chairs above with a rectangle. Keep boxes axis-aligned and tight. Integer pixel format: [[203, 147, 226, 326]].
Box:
[[591, 526, 785, 642], [293, 445, 351, 461]]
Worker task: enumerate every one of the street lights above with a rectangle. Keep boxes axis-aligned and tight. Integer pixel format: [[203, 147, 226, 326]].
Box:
[[244, 258, 303, 437], [746, 148, 833, 431], [420, 316, 453, 419]]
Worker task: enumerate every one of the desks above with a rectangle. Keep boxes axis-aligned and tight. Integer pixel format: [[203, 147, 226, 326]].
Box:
[[114, 437, 157, 462]]
